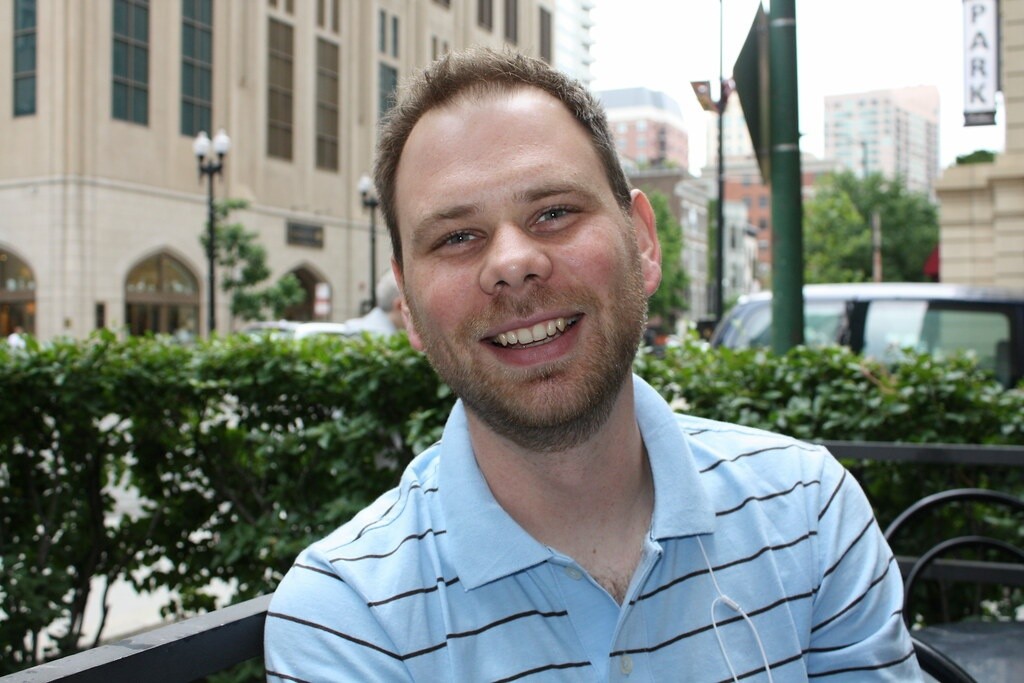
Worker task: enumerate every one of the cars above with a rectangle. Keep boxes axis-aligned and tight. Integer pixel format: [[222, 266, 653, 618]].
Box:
[[706, 283, 1024, 391]]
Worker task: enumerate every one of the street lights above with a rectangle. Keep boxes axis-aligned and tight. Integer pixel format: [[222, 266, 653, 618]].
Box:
[[356, 175, 382, 311], [190, 128, 232, 336]]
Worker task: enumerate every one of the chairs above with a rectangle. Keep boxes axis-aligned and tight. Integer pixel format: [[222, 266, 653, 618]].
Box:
[[996, 338, 1011, 385], [884, 487, 1024, 683]]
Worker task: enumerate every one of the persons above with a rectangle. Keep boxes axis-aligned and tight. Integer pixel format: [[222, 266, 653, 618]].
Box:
[[344, 269, 406, 340], [264, 46, 926, 683], [8, 325, 31, 361]]
